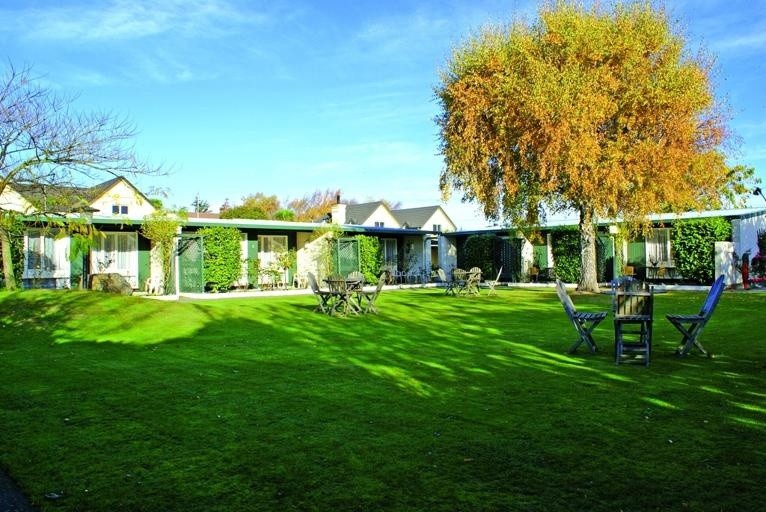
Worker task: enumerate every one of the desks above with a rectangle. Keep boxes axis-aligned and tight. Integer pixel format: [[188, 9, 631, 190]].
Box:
[[598, 288, 667, 362]]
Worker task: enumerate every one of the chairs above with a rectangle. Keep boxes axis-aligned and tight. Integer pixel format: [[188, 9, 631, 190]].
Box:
[[307, 270, 386, 319], [437, 265, 504, 297], [610, 275, 653, 369], [666, 273, 727, 358], [554, 278, 608, 354]]
